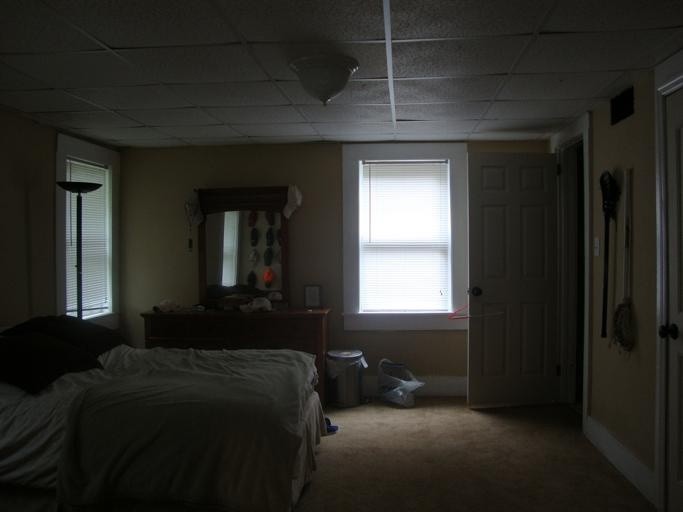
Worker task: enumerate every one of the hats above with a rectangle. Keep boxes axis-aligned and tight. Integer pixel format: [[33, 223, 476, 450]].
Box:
[[153, 300, 178, 312], [239, 298, 273, 313], [282, 184, 303, 220]]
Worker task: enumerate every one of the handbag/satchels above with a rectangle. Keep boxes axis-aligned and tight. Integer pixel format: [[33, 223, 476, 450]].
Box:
[[377, 359, 424, 406]]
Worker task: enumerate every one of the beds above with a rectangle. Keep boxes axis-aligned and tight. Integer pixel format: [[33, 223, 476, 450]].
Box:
[[1, 343, 327, 512]]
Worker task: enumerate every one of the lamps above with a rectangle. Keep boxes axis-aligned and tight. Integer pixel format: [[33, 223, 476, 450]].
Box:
[[56, 181, 102, 321], [289, 54, 360, 107]]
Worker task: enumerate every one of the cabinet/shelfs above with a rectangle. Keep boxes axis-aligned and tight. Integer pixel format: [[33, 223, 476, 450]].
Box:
[[140, 308, 331, 410]]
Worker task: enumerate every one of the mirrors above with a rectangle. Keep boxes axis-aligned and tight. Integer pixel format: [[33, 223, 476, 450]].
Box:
[[193, 186, 291, 311]]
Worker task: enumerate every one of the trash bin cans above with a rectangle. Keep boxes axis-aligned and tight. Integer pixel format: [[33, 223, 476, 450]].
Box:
[[326, 349, 363, 408], [381, 362, 405, 407]]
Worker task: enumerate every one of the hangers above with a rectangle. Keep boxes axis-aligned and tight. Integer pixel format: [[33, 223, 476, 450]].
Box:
[[448, 304, 468, 320]]
